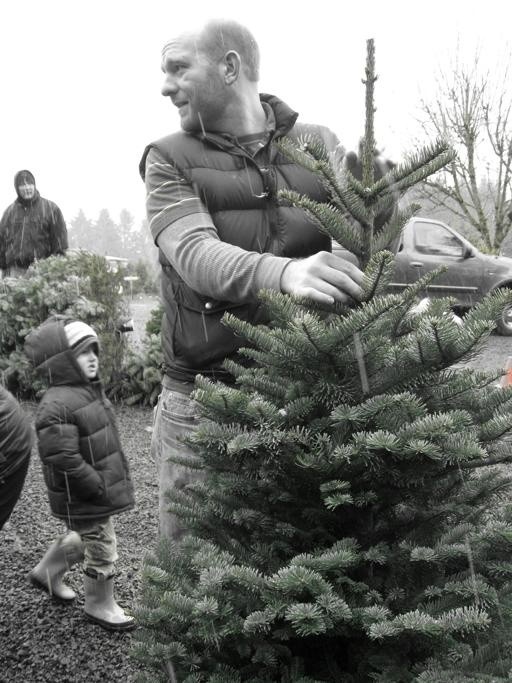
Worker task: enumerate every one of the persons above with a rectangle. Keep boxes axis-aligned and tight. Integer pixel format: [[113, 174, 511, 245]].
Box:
[[139, 17, 404, 562], [0, 170, 68, 280], [0, 382, 33, 531], [22, 315, 138, 630]]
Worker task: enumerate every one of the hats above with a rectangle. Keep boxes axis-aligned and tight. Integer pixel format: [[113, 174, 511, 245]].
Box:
[[64, 319, 100, 356]]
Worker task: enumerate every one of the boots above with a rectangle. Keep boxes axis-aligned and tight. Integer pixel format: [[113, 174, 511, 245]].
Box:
[[82, 565, 136, 631], [27, 537, 79, 602]]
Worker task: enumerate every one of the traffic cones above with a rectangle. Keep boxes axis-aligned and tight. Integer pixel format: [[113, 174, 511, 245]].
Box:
[[492, 356, 511, 388]]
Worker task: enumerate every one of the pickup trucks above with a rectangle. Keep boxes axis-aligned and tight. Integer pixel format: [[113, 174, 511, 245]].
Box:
[[329, 218, 511, 336]]
